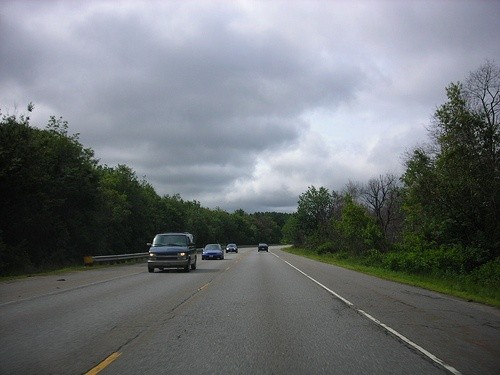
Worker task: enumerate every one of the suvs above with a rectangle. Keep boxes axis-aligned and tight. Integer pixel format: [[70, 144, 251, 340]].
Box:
[[258, 243, 268, 252]]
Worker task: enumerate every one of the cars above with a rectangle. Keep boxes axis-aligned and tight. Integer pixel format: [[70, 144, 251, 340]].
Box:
[[226, 244, 239, 253], [201, 244, 224, 260]]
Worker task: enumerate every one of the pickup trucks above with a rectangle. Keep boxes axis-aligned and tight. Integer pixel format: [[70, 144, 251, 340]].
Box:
[[146, 232, 197, 273]]
